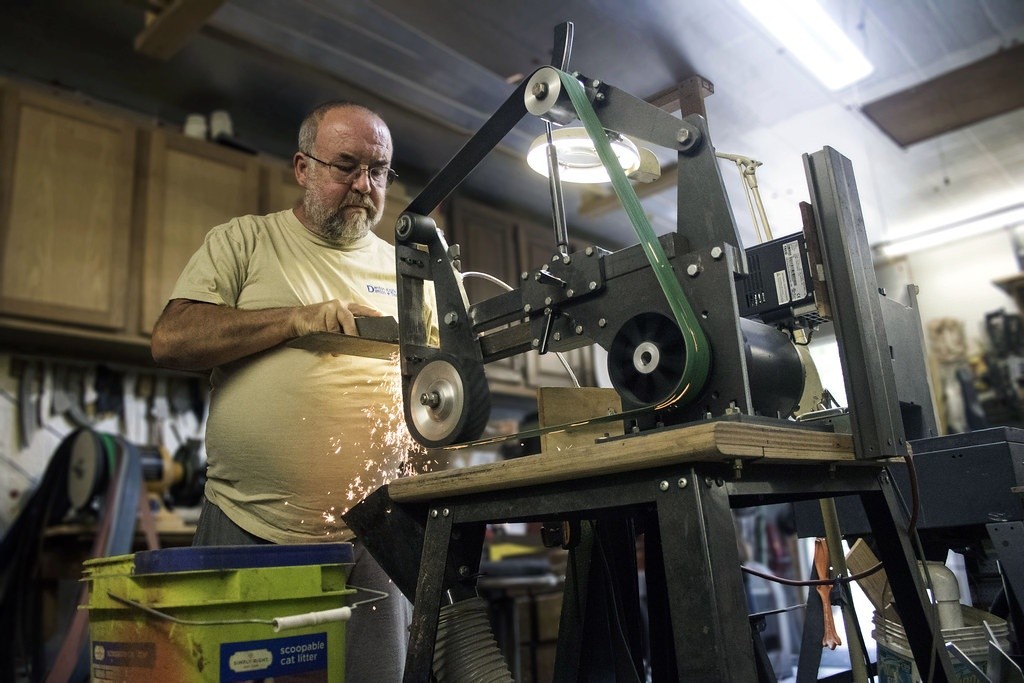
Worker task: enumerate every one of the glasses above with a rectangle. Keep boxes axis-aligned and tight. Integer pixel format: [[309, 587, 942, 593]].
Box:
[[303, 152, 400, 189]]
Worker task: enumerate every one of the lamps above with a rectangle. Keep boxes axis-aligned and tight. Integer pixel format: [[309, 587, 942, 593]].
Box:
[[527, 126, 641, 185]]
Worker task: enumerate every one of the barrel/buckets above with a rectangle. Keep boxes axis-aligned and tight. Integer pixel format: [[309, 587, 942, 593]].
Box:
[[872, 606, 1012, 683], [77, 540, 389, 683]]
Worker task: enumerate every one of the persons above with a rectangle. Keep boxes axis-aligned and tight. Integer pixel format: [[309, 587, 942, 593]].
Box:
[[152, 103, 440, 683]]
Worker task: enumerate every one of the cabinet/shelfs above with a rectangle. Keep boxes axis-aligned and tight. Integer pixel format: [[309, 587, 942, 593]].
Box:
[[1, 73, 599, 404]]
